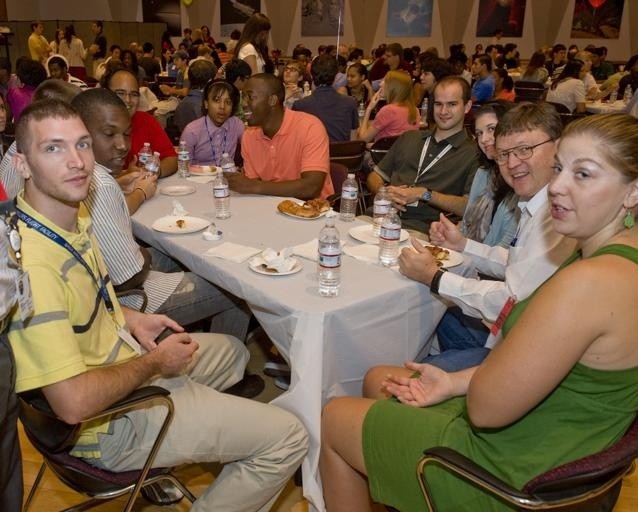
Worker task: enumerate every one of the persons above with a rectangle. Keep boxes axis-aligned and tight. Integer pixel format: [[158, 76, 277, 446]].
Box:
[[5, 98, 315, 511], [219, 72, 336, 346], [458, 96, 518, 249], [261, 75, 481, 394], [395, 101, 581, 376], [0, 215, 26, 510], [68, 87, 268, 396], [0, 1, 638, 240], [315, 111, 637, 512]]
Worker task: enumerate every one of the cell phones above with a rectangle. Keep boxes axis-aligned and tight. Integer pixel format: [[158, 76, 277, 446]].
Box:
[[154, 327, 176, 345]]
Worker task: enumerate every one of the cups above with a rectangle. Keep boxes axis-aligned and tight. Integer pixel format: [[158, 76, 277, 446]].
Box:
[[217, 70, 222, 78]]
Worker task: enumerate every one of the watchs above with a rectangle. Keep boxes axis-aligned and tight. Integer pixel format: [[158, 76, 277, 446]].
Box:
[[420, 186, 434, 203]]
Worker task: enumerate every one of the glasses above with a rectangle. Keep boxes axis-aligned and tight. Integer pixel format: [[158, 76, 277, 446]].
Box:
[[490, 137, 559, 166], [283, 65, 301, 72], [107, 88, 141, 99]]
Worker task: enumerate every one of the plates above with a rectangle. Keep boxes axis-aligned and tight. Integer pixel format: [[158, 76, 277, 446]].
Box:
[[159, 186, 196, 196], [248, 256, 305, 275], [152, 216, 211, 234], [400, 244, 463, 268], [189, 166, 222, 177], [277, 199, 327, 220], [349, 224, 410, 244]]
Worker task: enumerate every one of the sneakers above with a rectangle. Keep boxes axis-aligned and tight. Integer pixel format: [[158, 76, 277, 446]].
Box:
[[218, 371, 266, 400]]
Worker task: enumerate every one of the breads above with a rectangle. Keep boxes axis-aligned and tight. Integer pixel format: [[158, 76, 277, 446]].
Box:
[[425, 246, 449, 260], [278, 198, 329, 218]]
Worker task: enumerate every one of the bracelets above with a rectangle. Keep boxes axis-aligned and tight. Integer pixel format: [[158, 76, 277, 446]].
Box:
[[427, 265, 466, 294]]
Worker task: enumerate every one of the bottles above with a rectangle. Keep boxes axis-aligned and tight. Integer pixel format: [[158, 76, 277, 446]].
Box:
[[303, 81, 309, 97], [357, 103, 365, 128], [373, 186, 392, 238], [213, 171, 231, 220], [503, 64, 507, 70], [317, 220, 343, 297], [421, 98, 429, 125], [339, 173, 359, 222], [624, 84, 633, 107], [378, 209, 402, 267], [138, 142, 161, 177], [221, 153, 236, 173], [609, 89, 618, 105], [177, 141, 191, 178]]
